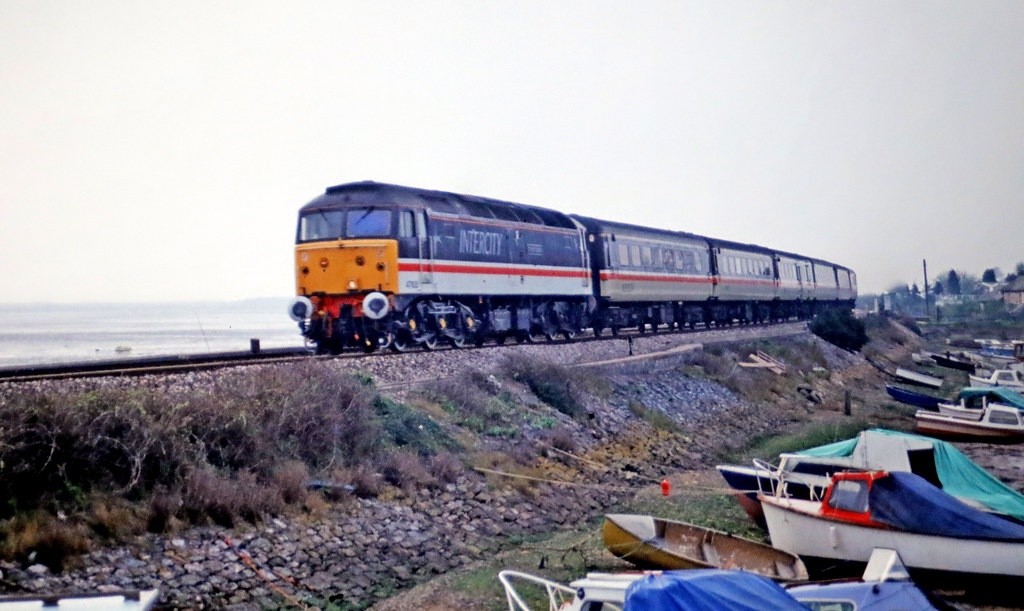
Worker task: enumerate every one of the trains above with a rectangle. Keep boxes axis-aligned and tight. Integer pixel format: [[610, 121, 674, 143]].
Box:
[[287, 179, 859, 352]]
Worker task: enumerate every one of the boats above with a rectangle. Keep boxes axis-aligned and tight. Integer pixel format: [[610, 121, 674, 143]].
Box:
[[600, 512, 813, 581], [885, 386, 1024, 414], [910, 402, 1024, 443], [713, 430, 1024, 537], [886, 369, 1024, 396], [911, 351, 1024, 368], [489, 546, 947, 611], [752, 456, 1023, 598]]
